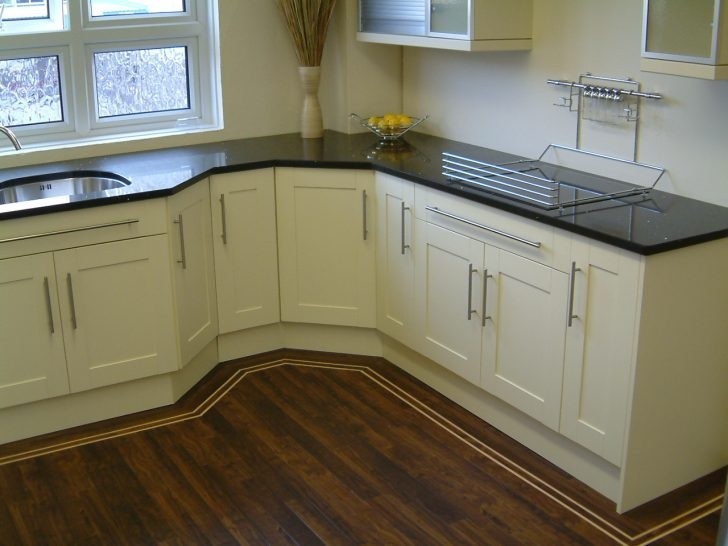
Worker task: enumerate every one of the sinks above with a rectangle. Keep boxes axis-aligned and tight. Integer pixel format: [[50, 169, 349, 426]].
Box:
[[0, 170, 132, 205]]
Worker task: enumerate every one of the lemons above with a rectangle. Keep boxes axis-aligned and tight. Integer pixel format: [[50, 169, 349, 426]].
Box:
[[368, 112, 414, 130]]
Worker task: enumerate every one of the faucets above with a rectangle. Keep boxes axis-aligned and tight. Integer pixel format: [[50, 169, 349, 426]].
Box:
[[0, 126, 23, 151]]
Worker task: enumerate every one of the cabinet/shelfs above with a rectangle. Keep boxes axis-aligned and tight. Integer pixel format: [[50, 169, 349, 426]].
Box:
[[358, 0, 536, 42], [167, 178, 220, 368], [375, 168, 415, 353], [558, 233, 642, 468], [274, 168, 375, 330], [412, 184, 570, 433], [641, 0, 728, 66], [208, 166, 281, 334], [0, 196, 179, 409]]
